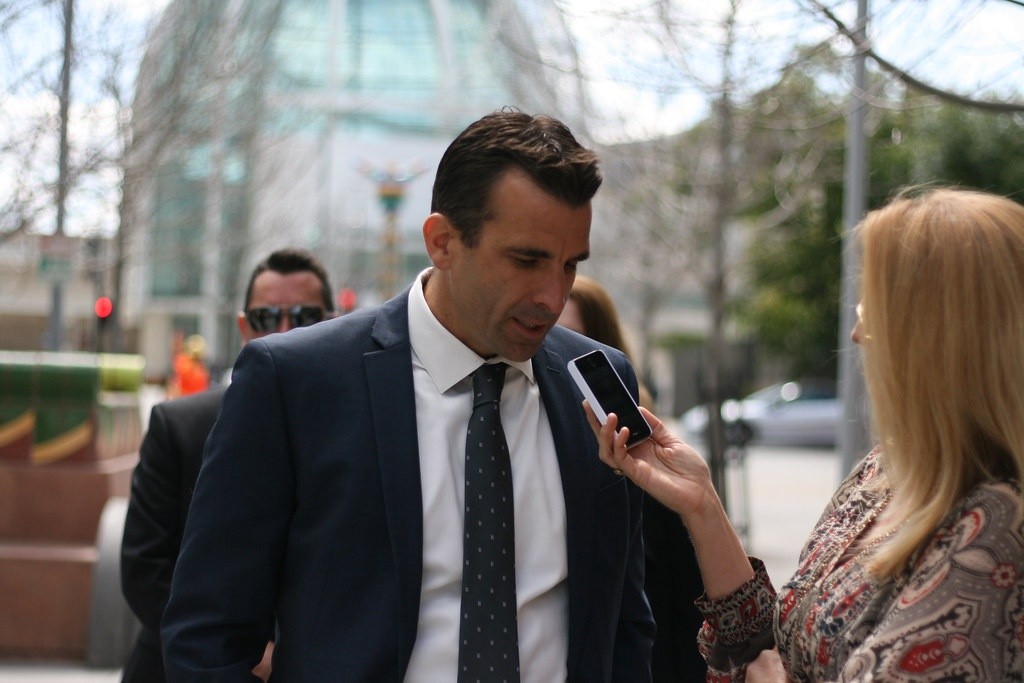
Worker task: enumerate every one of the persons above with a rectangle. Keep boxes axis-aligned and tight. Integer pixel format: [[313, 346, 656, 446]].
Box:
[[556, 280, 700, 683], [165, 108, 657, 681], [583, 184, 1024, 683], [118, 249, 334, 682]]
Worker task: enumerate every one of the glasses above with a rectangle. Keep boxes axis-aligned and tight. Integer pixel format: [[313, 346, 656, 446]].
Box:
[[245, 304, 335, 334]]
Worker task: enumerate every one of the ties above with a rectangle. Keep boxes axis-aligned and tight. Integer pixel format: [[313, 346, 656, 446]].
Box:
[[459, 361, 525, 683]]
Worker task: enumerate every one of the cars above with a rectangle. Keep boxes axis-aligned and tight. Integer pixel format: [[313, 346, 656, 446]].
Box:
[[681, 376, 842, 450]]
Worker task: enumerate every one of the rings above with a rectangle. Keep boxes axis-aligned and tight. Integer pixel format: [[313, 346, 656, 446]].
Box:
[[614, 469, 624, 475]]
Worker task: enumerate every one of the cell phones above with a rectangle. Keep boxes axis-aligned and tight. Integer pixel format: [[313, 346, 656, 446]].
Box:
[[567, 349, 652, 450]]
